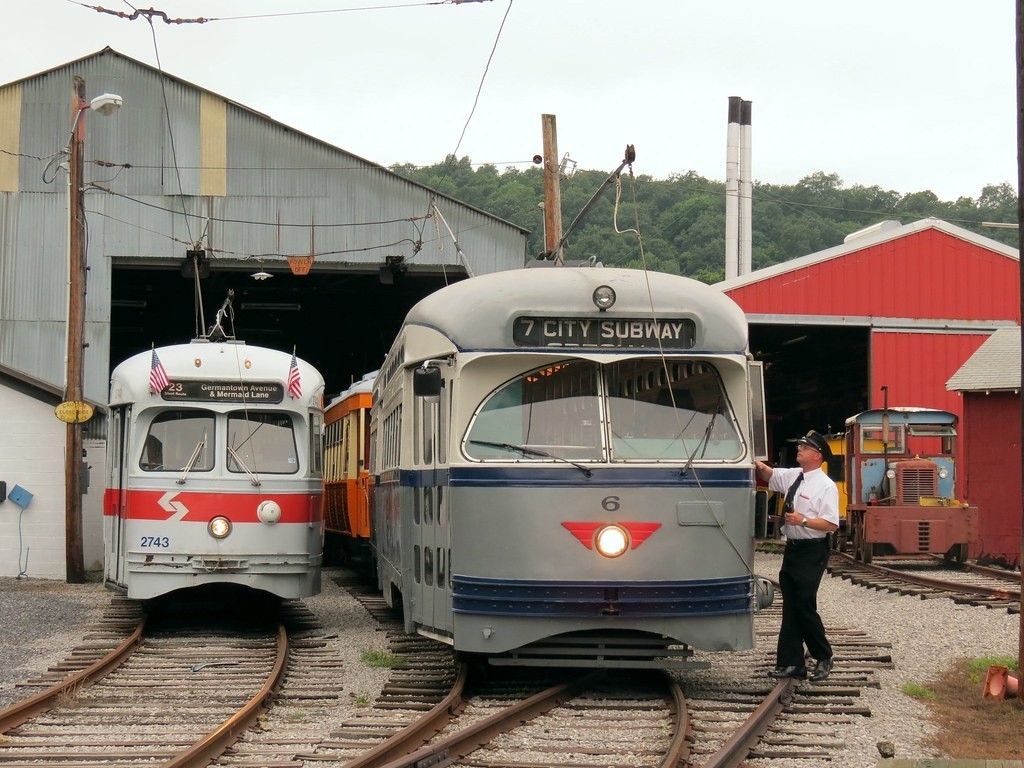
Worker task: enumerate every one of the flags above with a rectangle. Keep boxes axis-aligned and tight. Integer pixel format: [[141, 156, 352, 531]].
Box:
[[287, 346, 302, 399], [149, 346, 170, 395]]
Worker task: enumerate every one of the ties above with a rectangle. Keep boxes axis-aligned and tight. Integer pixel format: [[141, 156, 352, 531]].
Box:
[[781, 472, 804, 518]]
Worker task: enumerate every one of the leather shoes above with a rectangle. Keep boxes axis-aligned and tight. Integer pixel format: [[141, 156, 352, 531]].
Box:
[[808, 656, 833, 681], [767, 665, 808, 681]]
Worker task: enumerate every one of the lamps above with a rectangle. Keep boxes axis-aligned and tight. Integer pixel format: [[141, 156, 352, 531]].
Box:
[[250, 263, 274, 280]]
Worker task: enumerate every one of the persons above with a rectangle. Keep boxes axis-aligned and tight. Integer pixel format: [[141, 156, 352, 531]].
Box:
[[753, 431, 840, 682]]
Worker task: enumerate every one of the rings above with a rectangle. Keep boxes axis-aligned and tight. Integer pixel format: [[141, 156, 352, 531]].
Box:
[[786, 519, 788, 521]]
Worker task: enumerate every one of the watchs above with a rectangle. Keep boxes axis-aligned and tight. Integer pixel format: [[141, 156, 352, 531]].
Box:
[[801, 516, 808, 527]]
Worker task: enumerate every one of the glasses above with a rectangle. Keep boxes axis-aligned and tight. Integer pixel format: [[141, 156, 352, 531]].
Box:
[[798, 442, 819, 454]]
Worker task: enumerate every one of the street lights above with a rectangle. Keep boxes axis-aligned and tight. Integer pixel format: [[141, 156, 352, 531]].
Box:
[[41, 93, 124, 401]]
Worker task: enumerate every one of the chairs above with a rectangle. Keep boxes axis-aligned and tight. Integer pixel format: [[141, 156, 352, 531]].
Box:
[[650, 388, 697, 432]]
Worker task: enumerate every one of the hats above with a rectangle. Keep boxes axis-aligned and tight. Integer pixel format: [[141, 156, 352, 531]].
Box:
[[797, 430, 834, 462]]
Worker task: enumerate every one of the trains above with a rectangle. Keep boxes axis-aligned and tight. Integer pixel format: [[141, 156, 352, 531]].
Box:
[[102, 341, 327, 625], [324, 267, 763, 670], [757, 385, 980, 564]]
